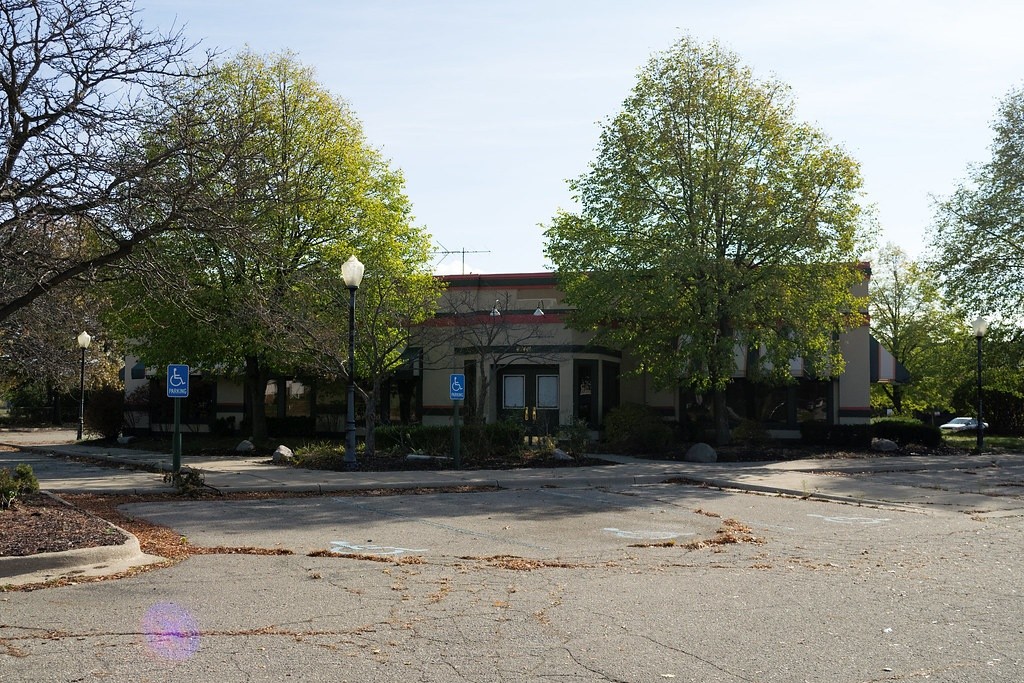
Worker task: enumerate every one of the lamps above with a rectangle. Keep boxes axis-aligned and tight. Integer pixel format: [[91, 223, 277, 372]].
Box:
[[534, 300, 547, 319], [490, 299, 505, 318]]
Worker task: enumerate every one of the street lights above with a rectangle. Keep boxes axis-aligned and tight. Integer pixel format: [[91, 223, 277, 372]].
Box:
[[338, 254, 366, 473], [974, 316, 989, 454], [77, 330, 91, 441]]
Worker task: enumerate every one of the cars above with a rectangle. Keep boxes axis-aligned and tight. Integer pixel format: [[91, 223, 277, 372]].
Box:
[[939, 417, 989, 432]]
